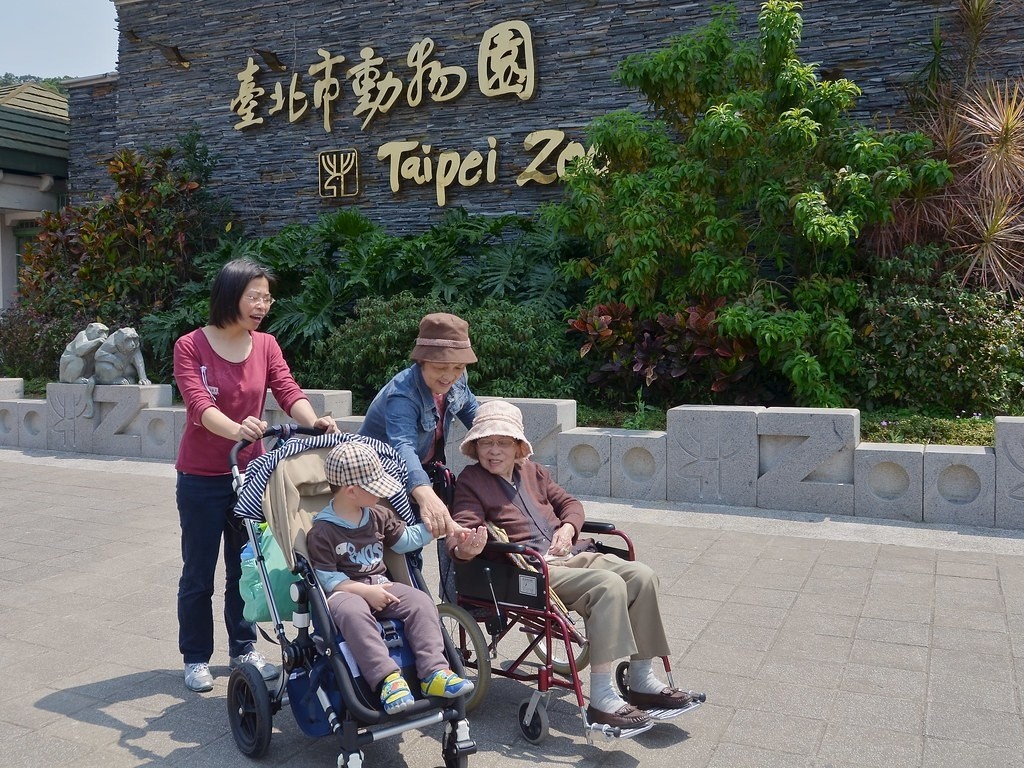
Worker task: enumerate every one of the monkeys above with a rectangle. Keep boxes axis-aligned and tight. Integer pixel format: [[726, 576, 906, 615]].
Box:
[[58, 322, 153, 386]]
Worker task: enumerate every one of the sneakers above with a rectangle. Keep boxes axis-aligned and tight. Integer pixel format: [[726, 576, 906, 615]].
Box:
[[184, 662, 215, 691], [420, 669, 474, 698], [379, 674, 414, 715], [230, 651, 280, 681]]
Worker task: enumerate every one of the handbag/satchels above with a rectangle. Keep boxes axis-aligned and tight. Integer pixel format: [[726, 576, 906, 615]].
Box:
[[239, 525, 311, 622]]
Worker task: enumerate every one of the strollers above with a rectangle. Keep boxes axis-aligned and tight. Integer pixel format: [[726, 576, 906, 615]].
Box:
[[226, 423, 479, 768]]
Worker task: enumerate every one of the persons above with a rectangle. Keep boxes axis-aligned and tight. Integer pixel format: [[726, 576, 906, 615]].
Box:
[[306, 442, 477, 714], [358, 312, 479, 571], [174, 257, 341, 693], [446, 400, 693, 729]]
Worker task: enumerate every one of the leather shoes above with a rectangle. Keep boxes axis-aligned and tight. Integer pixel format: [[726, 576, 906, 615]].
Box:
[[586, 704, 650, 728], [628, 687, 692, 708]]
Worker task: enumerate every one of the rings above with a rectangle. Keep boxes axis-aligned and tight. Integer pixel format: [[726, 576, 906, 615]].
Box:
[[471, 543, 478, 548], [564, 548, 567, 553]]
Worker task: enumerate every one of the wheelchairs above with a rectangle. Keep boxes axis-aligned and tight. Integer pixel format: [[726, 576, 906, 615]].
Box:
[[423, 460, 707, 747]]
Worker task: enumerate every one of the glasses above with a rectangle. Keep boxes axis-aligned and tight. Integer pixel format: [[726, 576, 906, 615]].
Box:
[[476, 438, 518, 451], [240, 295, 275, 306]]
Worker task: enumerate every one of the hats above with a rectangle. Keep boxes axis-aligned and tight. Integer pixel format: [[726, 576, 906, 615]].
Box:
[[410, 313, 478, 364], [324, 441, 403, 499], [459, 401, 534, 465]]
